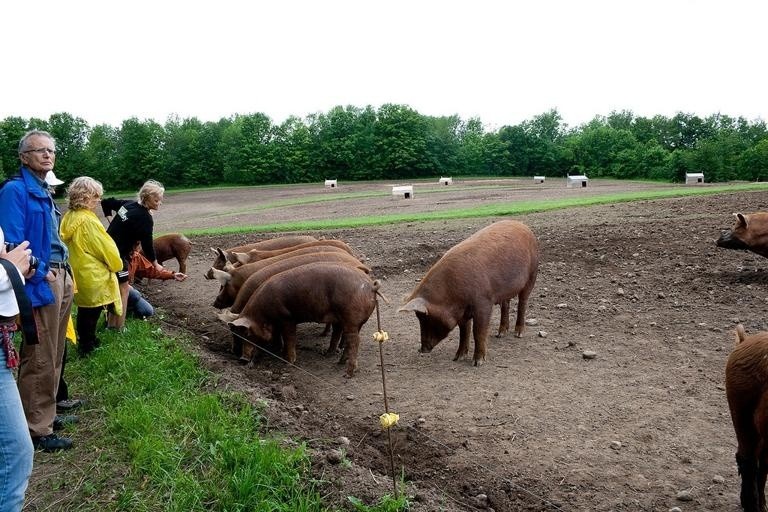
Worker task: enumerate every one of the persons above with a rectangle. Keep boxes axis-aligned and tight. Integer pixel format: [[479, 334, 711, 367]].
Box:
[[101, 179, 165, 331], [59, 176, 124, 354], [0, 226, 35, 512], [15, 170, 82, 414], [0, 129, 74, 452], [126, 241, 188, 319]]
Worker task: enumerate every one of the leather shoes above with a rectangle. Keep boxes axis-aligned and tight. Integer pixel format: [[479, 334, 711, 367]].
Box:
[[55, 400, 81, 411], [38, 434, 72, 450], [51, 414, 79, 430]]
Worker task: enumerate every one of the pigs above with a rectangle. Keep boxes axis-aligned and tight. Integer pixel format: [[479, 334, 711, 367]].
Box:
[[712, 209, 768, 260], [396, 217, 540, 368], [722, 321, 768, 512], [202, 234, 389, 380], [138, 233, 197, 281]]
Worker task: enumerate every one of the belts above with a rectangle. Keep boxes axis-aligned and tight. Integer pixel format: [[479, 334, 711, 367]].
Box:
[[49, 261, 69, 270]]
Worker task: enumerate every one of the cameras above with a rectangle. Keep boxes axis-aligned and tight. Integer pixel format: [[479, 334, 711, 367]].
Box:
[[5, 242, 39, 272]]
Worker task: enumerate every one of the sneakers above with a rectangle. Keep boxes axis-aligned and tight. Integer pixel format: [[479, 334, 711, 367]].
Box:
[[78, 337, 102, 353], [100, 321, 131, 334]]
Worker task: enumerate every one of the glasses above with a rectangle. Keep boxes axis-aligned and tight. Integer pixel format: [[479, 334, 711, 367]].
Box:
[[23, 147, 56, 154]]
